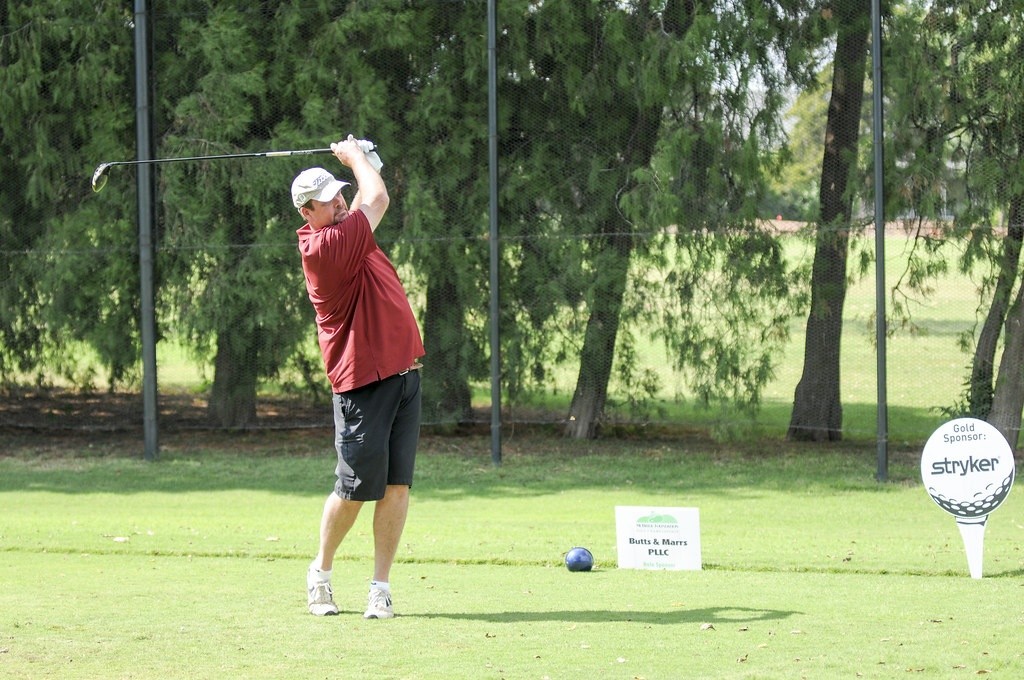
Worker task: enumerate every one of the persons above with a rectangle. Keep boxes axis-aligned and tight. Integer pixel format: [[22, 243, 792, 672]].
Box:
[[291, 134, 426, 618]]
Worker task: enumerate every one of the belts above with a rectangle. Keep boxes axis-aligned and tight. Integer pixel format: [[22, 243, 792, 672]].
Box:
[[398, 363, 424, 377]]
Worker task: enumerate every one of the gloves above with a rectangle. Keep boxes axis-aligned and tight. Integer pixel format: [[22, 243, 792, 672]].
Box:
[[348, 134, 383, 174]]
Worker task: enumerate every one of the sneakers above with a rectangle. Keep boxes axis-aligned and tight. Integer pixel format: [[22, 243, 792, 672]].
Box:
[[364, 586, 394, 620], [305, 563, 339, 616]]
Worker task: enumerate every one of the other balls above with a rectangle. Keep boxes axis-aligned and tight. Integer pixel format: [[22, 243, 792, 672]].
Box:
[[565, 546, 595, 573]]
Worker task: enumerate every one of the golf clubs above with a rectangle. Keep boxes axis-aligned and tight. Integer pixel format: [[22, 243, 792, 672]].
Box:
[[91, 144, 379, 193]]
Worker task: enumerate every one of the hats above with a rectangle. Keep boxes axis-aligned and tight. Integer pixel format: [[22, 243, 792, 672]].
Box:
[[290, 166, 352, 207]]
